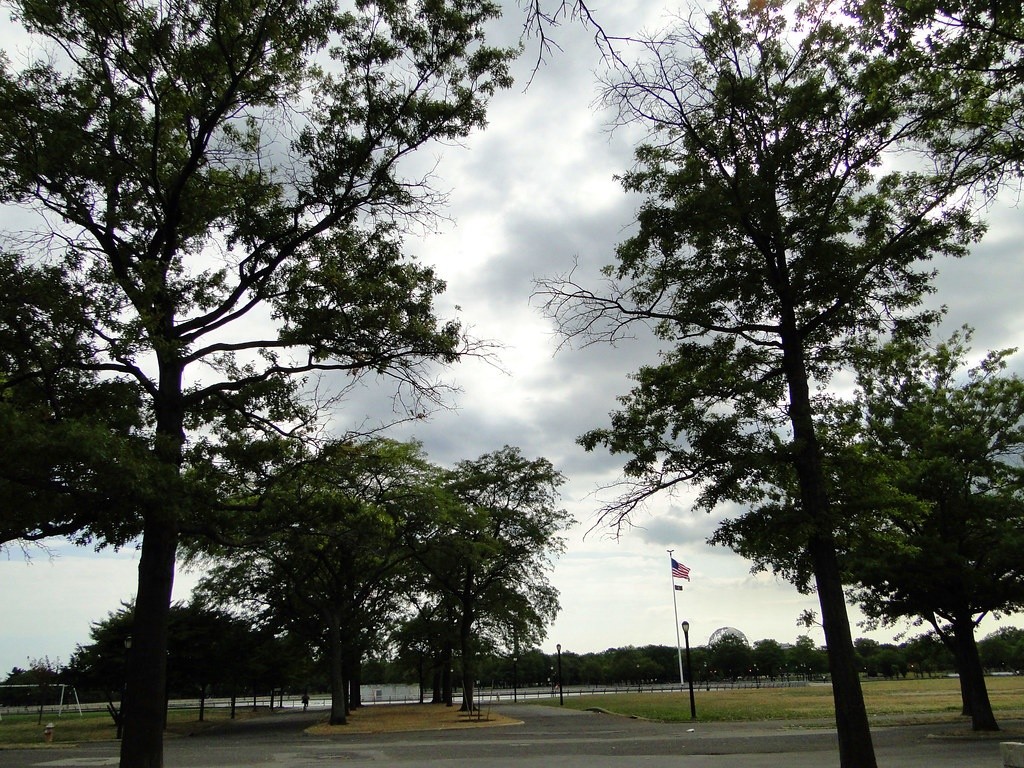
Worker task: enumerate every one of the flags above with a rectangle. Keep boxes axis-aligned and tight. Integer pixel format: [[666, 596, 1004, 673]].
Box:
[[671, 557, 690, 582], [675, 585, 682, 590]]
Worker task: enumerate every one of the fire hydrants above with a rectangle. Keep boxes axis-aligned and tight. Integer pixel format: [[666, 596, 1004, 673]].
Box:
[[44, 721, 55, 743]]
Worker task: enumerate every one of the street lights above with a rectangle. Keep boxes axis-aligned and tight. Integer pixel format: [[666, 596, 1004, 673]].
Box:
[[115, 635, 134, 738], [682, 620, 697, 719], [556, 644, 564, 706], [451, 668, 454, 702], [476, 680, 481, 705], [512, 653, 518, 702]]
